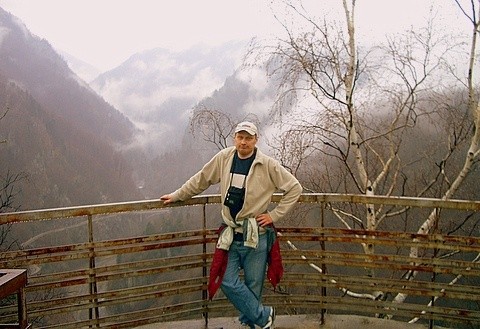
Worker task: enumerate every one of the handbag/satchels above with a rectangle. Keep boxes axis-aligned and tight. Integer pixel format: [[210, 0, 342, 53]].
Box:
[[223, 186, 246, 208]]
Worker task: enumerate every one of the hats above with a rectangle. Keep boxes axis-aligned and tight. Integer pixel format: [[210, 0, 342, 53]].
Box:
[[235, 121, 258, 137]]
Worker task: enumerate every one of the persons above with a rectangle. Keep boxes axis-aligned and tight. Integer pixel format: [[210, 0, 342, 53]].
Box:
[[159, 122, 303, 329]]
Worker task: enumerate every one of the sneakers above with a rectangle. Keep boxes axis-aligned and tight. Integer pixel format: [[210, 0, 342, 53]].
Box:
[[254, 306, 275, 329]]
